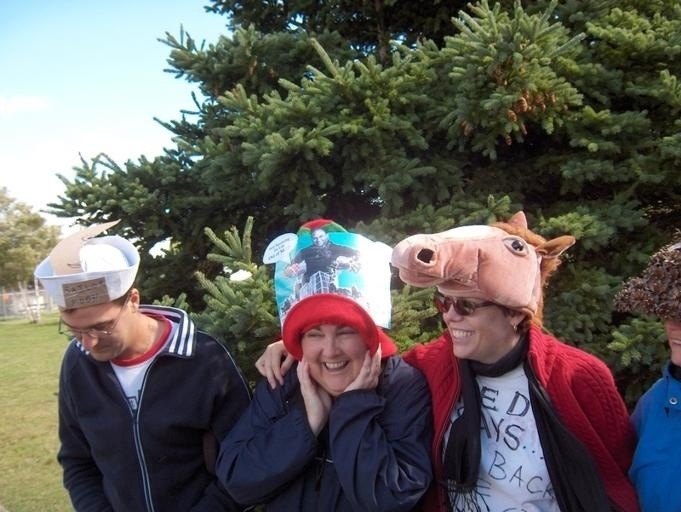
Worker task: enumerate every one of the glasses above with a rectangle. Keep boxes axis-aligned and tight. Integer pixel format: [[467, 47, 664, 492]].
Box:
[[59, 300, 128, 339], [433, 288, 496, 316]]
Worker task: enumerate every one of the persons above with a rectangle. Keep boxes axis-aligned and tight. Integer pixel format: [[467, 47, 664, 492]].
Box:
[[253, 289, 640, 511], [625, 315, 681, 511], [282, 227, 364, 286], [35, 217, 253, 510], [218, 317, 433, 511]]
